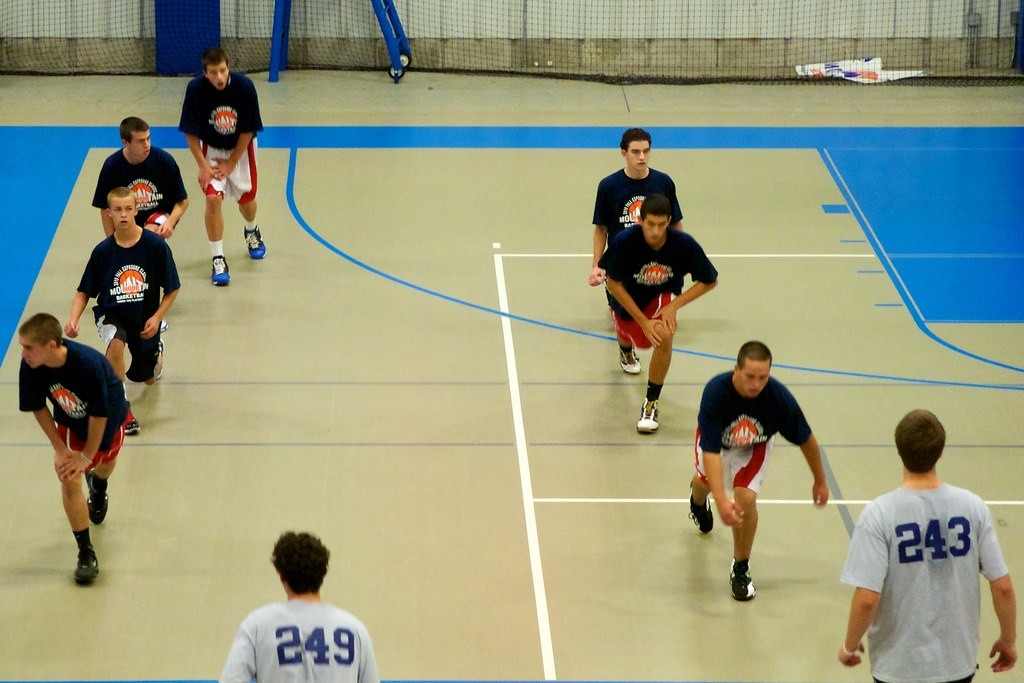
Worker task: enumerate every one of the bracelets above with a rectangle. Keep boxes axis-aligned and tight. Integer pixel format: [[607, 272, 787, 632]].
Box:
[[79, 452, 93, 465], [842, 642, 858, 656]]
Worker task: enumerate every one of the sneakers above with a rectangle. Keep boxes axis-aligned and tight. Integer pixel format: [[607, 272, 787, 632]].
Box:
[[637, 397, 659, 433], [211, 256, 230, 286], [730, 557, 757, 601], [154, 339, 164, 379], [75, 544, 98, 584], [85, 467, 108, 524], [688, 481, 713, 533], [158, 317, 168, 332], [121, 400, 140, 434], [620, 342, 641, 374], [244, 225, 266, 258]]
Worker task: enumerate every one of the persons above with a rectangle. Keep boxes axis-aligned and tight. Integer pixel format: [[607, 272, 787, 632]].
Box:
[[597, 192, 719, 435], [92, 116, 188, 332], [688, 342, 829, 601], [64, 187, 164, 434], [19, 313, 128, 584], [178, 49, 266, 286], [838, 408, 1017, 683], [587, 128, 683, 305], [221, 530, 382, 683]]
